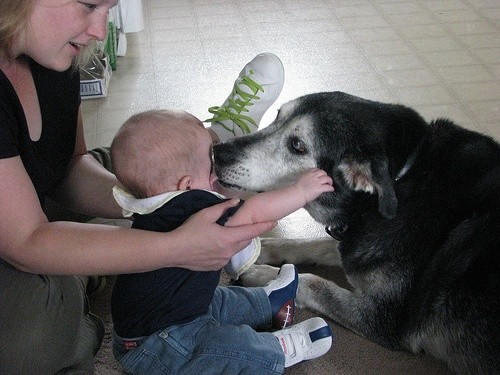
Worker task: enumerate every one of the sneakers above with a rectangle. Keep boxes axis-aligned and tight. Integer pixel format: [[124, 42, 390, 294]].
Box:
[[83, 215, 132, 229], [262, 263, 299, 328], [272, 316, 333, 368], [200, 52, 286, 143]]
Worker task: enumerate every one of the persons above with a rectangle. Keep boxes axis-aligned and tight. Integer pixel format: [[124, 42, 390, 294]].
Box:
[[0, 0, 285, 375], [110, 108, 335, 375]]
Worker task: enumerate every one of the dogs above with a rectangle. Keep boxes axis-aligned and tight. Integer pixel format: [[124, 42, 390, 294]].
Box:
[[213, 90, 500, 375]]
